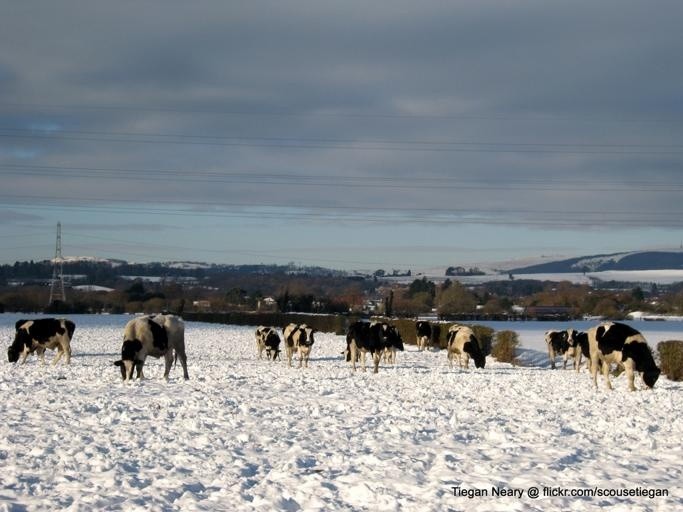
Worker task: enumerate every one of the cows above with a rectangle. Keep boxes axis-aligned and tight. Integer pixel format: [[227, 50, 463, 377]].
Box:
[[114, 314, 189, 380], [446, 324, 486, 369], [340, 321, 404, 373], [544, 321, 661, 392], [255, 325, 281, 362], [416, 321, 441, 351], [282, 322, 319, 369], [6, 316, 76, 367]]
